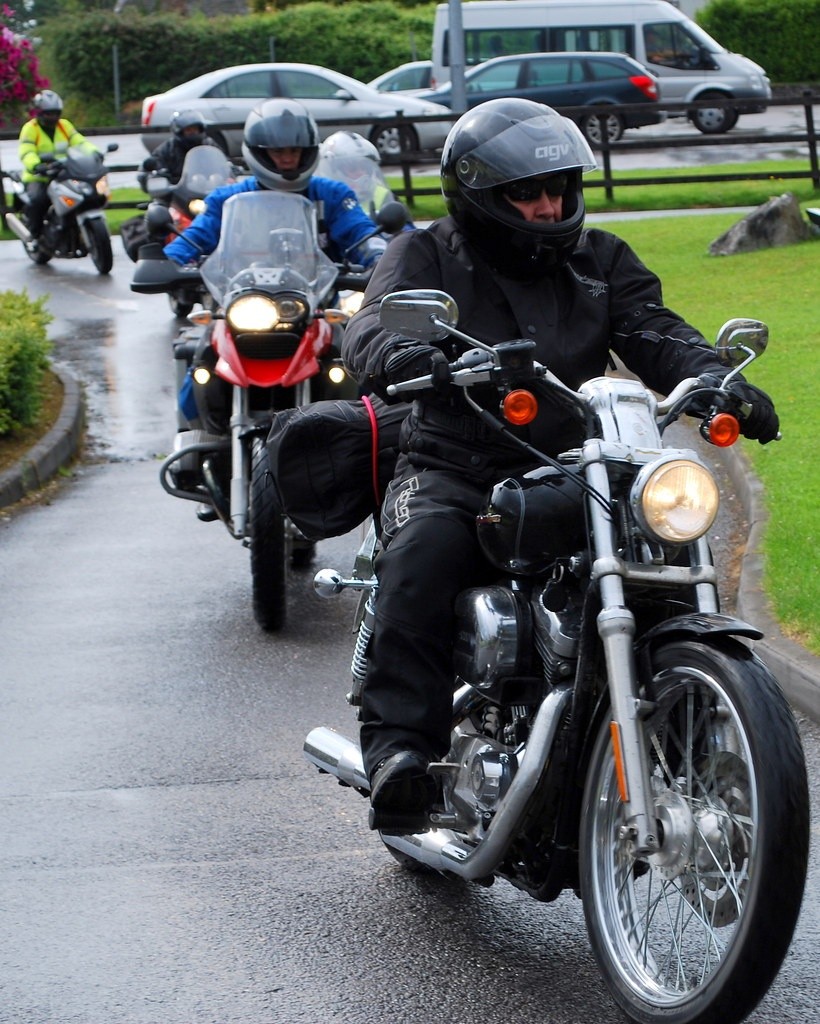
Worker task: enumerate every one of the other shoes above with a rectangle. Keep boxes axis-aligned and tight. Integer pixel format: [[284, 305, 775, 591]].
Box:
[[27, 239, 38, 252], [370, 750, 434, 837], [196, 503, 215, 522]]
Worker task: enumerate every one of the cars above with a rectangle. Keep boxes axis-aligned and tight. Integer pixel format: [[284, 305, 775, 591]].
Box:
[[139, 61, 455, 168], [366, 59, 473, 99]]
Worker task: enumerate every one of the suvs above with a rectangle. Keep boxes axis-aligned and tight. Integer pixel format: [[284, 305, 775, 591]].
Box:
[[413, 50, 670, 148]]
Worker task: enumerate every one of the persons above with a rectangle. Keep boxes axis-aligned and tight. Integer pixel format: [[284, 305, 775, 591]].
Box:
[[137, 107, 242, 231], [18, 89, 104, 252], [645, 32, 683, 64], [313, 129, 417, 243], [160, 97, 393, 521], [341, 98, 780, 837]]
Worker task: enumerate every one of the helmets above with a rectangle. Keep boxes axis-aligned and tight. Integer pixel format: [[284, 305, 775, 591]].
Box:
[[32, 89, 62, 128], [441, 97, 595, 283], [319, 131, 382, 203], [237, 100, 321, 196], [170, 110, 208, 148]]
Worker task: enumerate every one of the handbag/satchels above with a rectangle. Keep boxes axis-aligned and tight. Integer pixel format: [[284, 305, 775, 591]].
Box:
[[268, 392, 414, 543]]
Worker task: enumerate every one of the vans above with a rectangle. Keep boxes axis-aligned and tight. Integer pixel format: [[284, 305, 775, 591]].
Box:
[[430, 0, 773, 135]]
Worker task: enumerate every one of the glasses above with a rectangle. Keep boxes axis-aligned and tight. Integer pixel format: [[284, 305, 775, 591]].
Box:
[[502, 172, 573, 202]]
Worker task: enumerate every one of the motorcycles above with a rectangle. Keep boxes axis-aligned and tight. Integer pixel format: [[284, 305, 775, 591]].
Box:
[[1, 143, 120, 274], [301, 286, 813, 1024], [119, 146, 248, 261], [134, 203, 410, 635]]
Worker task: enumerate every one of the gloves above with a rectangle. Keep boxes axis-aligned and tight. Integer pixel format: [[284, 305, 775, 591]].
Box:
[[35, 164, 49, 176], [382, 340, 453, 400], [700, 378, 781, 447]]
